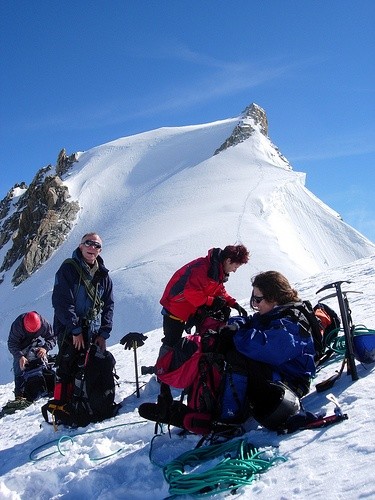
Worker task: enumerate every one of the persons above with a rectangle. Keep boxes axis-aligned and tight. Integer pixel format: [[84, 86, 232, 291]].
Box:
[[51, 231, 116, 401], [7, 311, 56, 398], [215, 271, 317, 437], [155, 243, 250, 382]]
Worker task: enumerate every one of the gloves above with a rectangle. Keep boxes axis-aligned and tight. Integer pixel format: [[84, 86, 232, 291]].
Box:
[[233, 302, 247, 318]]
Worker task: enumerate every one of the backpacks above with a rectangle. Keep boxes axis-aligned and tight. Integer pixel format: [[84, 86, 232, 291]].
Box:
[[23, 347, 55, 402], [314, 304, 341, 356], [41, 344, 119, 430]]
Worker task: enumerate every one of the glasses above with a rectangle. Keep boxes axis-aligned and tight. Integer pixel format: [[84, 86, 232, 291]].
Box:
[[253, 296, 264, 303], [81, 240, 102, 249]]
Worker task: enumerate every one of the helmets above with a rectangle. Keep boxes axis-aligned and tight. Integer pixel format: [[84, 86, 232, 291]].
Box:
[[352, 334, 375, 363], [140, 336, 201, 389], [24, 312, 41, 332]]
